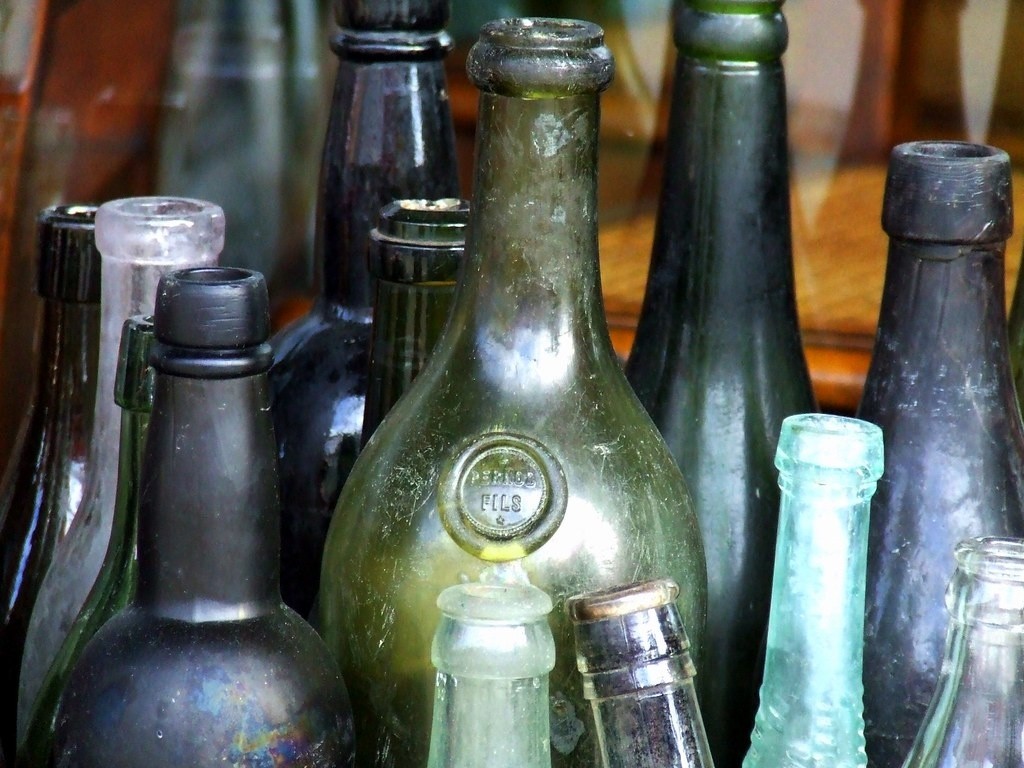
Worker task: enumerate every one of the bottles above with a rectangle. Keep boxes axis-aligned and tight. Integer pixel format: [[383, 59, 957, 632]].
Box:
[[0, 0, 1024, 768]]
